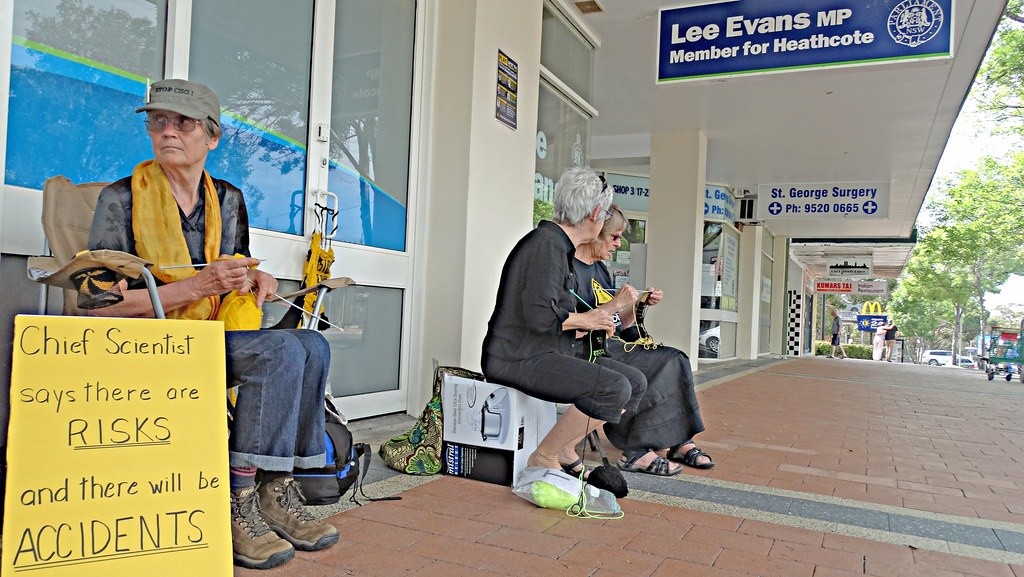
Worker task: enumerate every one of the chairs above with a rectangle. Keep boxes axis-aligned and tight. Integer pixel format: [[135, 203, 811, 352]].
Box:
[[27, 172, 165, 320]]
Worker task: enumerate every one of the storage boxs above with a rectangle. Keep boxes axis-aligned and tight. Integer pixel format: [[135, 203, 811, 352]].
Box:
[[442, 369, 558, 487]]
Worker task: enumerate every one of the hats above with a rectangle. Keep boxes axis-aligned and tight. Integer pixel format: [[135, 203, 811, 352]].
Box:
[[135, 79, 220, 127]]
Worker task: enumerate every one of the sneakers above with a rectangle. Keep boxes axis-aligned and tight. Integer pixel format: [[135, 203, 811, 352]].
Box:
[[230, 482, 295, 569], [258, 476, 339, 552]]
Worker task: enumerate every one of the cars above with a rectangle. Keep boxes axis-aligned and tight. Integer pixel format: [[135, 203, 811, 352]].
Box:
[[947, 357, 976, 370]]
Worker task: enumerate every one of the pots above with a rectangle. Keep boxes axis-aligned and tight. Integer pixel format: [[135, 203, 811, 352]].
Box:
[[481, 400, 501, 441]]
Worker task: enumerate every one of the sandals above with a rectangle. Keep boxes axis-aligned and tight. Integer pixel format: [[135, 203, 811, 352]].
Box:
[[617, 449, 683, 476], [666, 440, 715, 470]]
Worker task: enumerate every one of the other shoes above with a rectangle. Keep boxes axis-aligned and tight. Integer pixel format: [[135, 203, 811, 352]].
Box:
[[886, 358, 892, 362], [874, 359, 880, 361], [826, 355, 834, 359], [840, 356, 847, 359]]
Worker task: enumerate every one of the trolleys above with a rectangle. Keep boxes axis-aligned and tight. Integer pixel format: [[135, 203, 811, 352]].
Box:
[[987, 327, 1024, 383]]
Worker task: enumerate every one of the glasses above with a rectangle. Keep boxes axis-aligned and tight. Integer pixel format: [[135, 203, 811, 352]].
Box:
[[602, 209, 613, 221], [610, 233, 622, 241], [143, 115, 202, 132]]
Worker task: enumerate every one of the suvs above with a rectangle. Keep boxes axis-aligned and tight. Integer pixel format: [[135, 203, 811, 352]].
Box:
[[921, 349, 960, 366]]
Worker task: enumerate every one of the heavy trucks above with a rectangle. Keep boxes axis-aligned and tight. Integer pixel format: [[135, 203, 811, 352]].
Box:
[[976, 335, 1015, 373]]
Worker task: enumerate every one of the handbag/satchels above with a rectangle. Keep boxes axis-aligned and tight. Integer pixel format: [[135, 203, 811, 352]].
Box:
[[292, 390, 403, 507], [378, 365, 485, 476]]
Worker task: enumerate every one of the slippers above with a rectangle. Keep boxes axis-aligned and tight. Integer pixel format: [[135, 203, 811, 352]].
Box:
[[559, 456, 593, 481]]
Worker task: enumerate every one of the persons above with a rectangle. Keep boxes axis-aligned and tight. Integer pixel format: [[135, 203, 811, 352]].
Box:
[[74, 80, 339, 570], [873, 320, 899, 362], [481, 166, 714, 483], [826, 309, 848, 358]]
